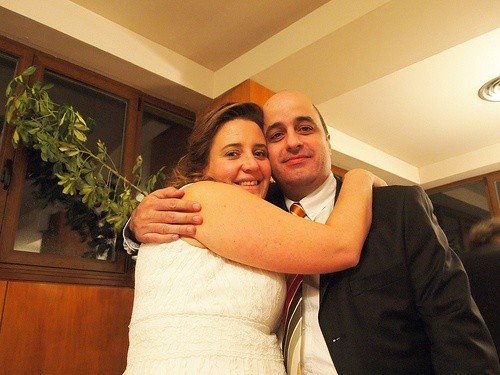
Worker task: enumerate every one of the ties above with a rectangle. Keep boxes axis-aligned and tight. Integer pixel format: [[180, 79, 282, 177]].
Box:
[[282, 201, 309, 375]]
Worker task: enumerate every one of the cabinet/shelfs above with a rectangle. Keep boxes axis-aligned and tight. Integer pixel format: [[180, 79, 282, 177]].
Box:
[[0, 34, 196, 375]]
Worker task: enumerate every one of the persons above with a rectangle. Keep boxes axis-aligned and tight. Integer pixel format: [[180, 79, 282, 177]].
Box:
[[122, 101, 388, 375], [120, 89, 500, 375], [463, 216, 500, 351]]
